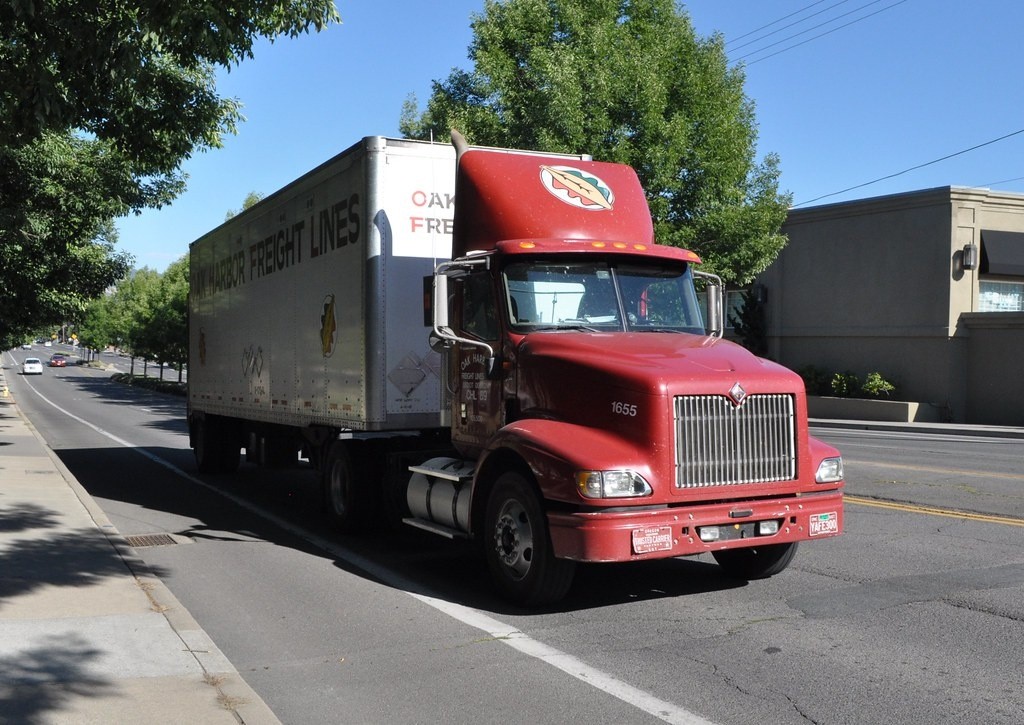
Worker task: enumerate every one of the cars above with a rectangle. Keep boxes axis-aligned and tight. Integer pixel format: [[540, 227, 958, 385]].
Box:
[[22, 358, 45, 375], [21, 343, 32, 350], [49, 353, 67, 368]]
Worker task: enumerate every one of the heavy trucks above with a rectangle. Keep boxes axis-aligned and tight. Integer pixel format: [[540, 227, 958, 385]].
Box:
[[188, 123, 845, 614]]
